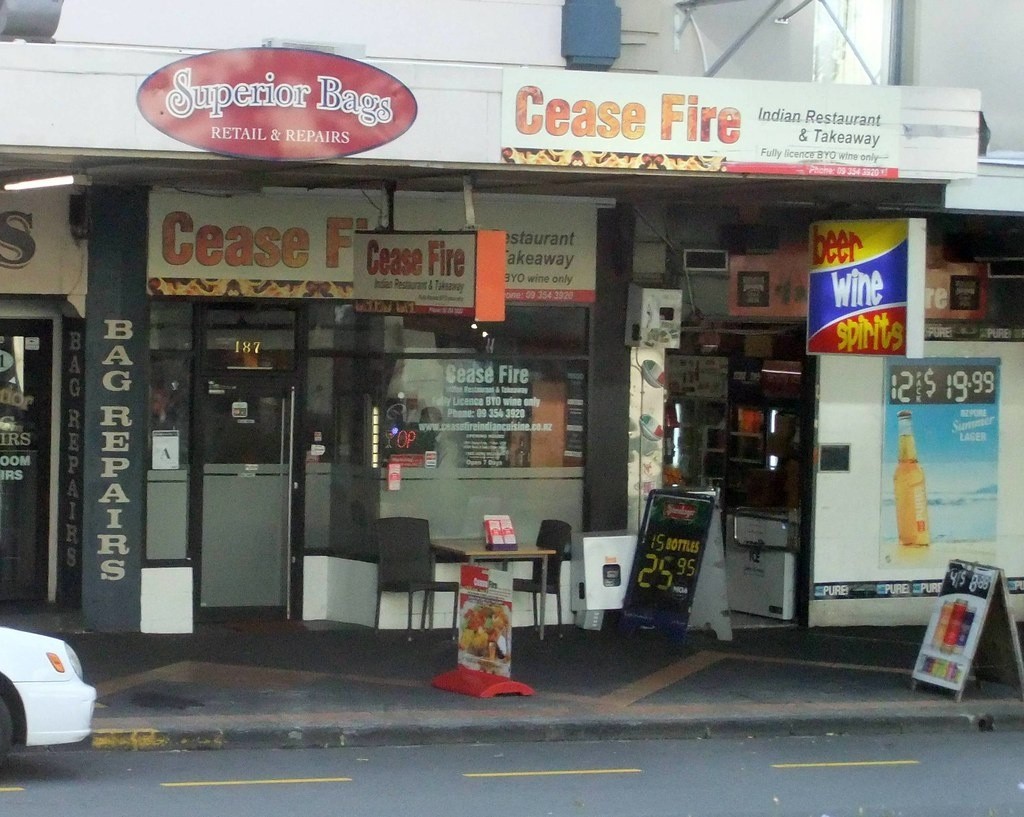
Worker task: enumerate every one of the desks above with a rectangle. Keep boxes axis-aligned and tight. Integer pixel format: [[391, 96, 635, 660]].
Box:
[[429, 537, 557, 641]]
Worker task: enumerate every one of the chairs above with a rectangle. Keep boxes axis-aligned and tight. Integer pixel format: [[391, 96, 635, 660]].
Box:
[[513, 519, 572, 641], [714, 471, 789, 508], [373, 517, 460, 643]]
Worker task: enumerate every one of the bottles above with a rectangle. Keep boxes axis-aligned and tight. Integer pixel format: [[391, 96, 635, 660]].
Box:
[[894, 410, 930, 548], [917, 598, 977, 684]]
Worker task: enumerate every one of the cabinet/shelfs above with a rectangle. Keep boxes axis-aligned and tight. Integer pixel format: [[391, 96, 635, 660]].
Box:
[[664, 322, 807, 513]]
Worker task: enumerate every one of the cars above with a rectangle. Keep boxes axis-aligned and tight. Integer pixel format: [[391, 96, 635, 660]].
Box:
[[0, 621, 100, 760]]
